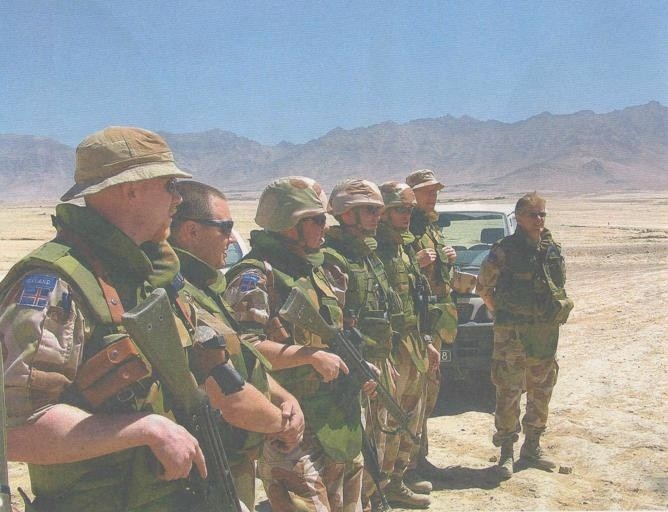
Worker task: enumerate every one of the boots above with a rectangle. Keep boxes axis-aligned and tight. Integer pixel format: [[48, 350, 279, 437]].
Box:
[[383, 470, 433, 509], [416, 457, 448, 480]]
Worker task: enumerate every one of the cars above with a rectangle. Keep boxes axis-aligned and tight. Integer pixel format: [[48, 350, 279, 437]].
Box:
[[194, 223, 252, 275]]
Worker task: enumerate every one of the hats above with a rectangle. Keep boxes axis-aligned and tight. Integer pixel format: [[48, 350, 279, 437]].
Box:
[[405, 169, 444, 191], [60, 126, 191, 201]]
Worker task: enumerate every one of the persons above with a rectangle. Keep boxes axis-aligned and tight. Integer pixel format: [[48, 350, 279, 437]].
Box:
[[0, 124, 209, 511], [473, 191, 574, 477], [403, 168, 460, 478], [378, 181, 442, 511], [221, 173, 384, 512], [322, 176, 402, 511], [173, 179, 305, 511]]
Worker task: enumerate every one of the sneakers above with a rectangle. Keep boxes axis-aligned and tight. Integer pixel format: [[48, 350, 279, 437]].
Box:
[[497, 456, 514, 479], [520, 446, 556, 469]]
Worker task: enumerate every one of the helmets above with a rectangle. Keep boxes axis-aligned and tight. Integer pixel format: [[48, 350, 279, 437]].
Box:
[[255, 176, 332, 233], [327, 179, 385, 217], [449, 271, 477, 293], [378, 182, 417, 213]]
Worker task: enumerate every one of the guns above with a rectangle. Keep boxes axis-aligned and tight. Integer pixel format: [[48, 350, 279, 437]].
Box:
[[120, 287, 242, 512], [279, 286, 421, 445], [358, 424, 392, 512]]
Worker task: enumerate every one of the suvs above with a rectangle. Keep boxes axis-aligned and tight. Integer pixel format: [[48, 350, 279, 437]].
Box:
[[379, 198, 528, 399]]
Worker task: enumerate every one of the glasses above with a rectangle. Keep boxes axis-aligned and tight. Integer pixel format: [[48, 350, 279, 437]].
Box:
[[184, 216, 232, 235], [305, 216, 327, 226], [529, 213, 545, 217], [395, 206, 413, 214], [167, 177, 176, 191], [362, 205, 381, 215]]
